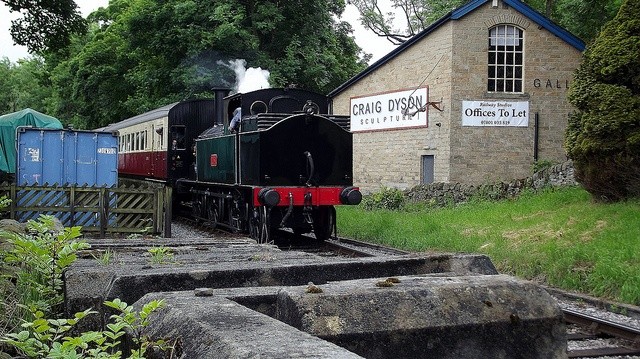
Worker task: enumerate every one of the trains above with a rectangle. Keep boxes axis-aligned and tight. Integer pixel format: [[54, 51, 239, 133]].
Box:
[[92, 88, 362, 244]]
